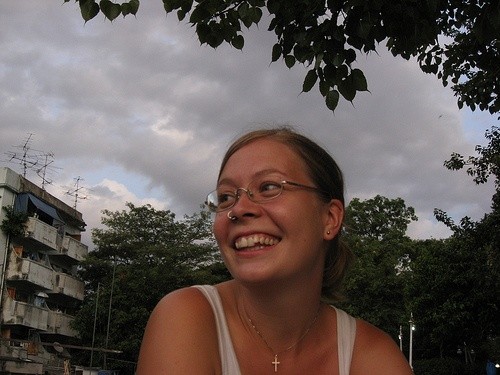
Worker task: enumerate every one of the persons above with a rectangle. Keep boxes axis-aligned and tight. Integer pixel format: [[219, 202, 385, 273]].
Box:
[[135, 128, 414, 375]]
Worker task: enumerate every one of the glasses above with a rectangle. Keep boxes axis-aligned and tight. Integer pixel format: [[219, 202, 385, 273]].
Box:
[[205, 175, 334, 213]]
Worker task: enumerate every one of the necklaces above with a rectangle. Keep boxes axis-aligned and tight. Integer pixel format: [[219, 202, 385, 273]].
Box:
[[241, 298, 321, 371]]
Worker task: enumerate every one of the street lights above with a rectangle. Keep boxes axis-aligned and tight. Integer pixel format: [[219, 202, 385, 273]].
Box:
[[457, 344, 475, 366], [398, 325, 403, 352], [408, 312, 415, 375], [103, 254, 130, 372]]
